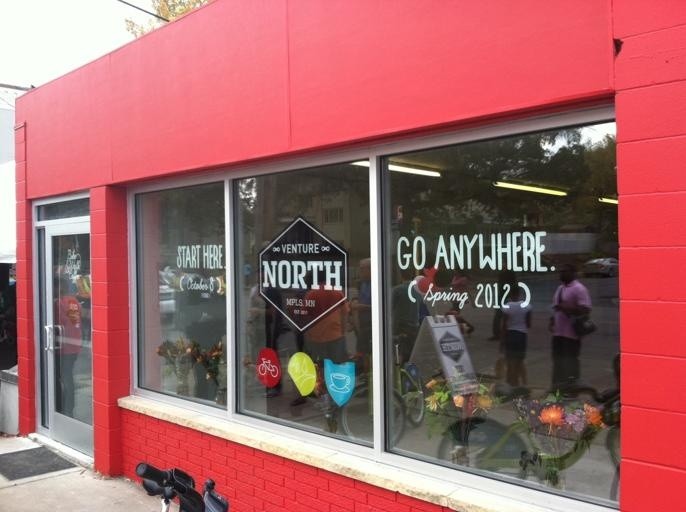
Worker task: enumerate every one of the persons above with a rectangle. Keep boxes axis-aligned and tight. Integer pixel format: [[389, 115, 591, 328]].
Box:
[[390, 258, 474, 363], [548, 262, 593, 400], [54, 278, 83, 418], [248, 258, 371, 416], [488, 258, 518, 341], [168, 275, 222, 399], [502, 285, 533, 387]]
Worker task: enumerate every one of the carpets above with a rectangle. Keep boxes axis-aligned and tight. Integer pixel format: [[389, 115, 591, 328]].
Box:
[[0, 446, 78, 482]]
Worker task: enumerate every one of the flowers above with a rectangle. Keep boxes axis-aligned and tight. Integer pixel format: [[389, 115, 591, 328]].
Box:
[[153, 330, 226, 396], [399, 378, 607, 488]]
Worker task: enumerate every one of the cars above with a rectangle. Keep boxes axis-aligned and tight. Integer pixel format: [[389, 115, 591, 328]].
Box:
[[582, 257, 618, 277]]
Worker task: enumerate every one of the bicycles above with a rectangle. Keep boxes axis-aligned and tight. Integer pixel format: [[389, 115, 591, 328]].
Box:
[[437, 351, 620, 501], [340, 335, 425, 447]]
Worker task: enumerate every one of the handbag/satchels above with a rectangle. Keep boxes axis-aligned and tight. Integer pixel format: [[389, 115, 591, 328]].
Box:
[[575, 314, 598, 337]]
